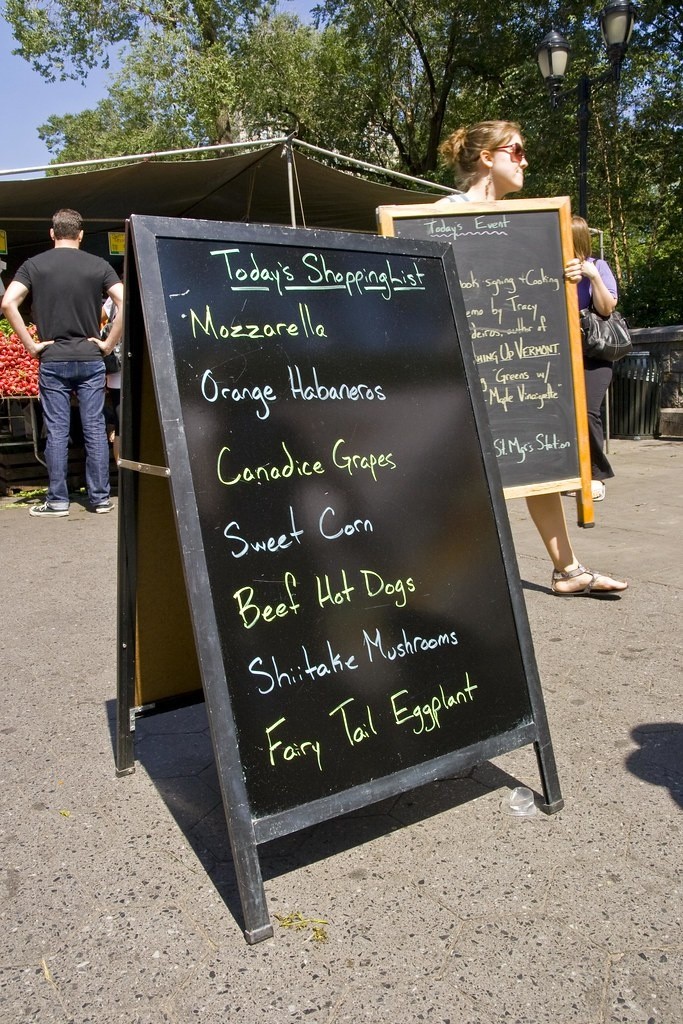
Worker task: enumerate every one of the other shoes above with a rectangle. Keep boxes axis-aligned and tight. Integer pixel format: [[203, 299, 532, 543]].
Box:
[[592, 481, 606, 501], [566, 491, 577, 497]]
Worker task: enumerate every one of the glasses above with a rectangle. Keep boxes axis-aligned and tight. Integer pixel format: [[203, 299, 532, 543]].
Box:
[[473, 143, 528, 162]]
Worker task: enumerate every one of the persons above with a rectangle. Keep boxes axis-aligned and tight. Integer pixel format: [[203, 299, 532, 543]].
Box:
[[434, 120, 627, 595], [0, 209, 124, 517], [560, 215, 618, 501]]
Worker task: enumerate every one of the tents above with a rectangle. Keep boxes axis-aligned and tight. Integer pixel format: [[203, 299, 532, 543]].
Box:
[[0, 131, 465, 235]]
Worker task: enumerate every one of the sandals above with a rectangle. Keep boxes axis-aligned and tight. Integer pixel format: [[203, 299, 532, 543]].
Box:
[[551, 563, 629, 596]]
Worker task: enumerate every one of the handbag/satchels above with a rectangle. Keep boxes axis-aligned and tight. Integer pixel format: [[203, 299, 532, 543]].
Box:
[[580, 307, 633, 361]]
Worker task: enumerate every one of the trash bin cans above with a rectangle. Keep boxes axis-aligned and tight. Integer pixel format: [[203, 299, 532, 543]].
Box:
[[612, 355, 663, 441]]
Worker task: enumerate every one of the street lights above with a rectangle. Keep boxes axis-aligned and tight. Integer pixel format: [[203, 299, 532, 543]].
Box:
[[533, 0, 638, 222]]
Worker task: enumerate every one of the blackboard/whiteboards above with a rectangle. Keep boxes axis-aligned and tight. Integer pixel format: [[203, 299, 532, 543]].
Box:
[[114, 214, 546, 845], [378, 196, 591, 499]]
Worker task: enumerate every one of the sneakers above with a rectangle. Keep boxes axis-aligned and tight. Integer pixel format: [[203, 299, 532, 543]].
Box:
[[96, 500, 115, 513], [29, 502, 70, 517]]
